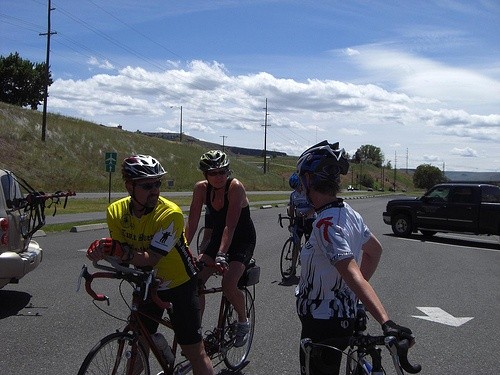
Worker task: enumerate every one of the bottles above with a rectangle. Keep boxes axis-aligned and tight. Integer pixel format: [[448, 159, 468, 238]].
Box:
[[154, 331, 175, 364]]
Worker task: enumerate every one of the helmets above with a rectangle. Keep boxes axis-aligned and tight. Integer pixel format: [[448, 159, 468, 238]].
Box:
[[121, 155, 167, 181], [296, 140, 350, 177], [199, 149, 229, 172], [288, 173, 300, 189]]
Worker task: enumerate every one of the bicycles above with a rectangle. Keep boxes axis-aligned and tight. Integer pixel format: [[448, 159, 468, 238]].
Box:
[[278, 211, 308, 281], [75, 251, 261, 375], [301, 301, 423, 375]]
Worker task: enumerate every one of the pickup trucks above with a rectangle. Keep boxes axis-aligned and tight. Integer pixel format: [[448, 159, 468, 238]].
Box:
[[383, 181, 500, 238]]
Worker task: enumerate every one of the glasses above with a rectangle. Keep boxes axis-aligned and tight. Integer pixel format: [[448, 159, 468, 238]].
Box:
[[130, 179, 161, 190], [206, 168, 228, 176]]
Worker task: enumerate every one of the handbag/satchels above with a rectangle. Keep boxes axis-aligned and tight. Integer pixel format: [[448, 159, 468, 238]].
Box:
[[238, 258, 260, 286]]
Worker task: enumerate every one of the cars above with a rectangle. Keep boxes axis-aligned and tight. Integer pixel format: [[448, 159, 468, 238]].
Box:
[[368, 189, 373, 192], [347, 185, 354, 191]]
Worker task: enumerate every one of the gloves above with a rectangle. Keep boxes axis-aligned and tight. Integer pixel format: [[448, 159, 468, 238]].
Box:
[[382, 320, 416, 348]]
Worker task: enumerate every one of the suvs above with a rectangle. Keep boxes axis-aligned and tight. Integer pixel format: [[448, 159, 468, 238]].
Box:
[[0, 166, 42, 291]]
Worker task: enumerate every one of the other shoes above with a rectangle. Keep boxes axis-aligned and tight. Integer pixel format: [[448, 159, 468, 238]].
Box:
[[283, 266, 296, 276]]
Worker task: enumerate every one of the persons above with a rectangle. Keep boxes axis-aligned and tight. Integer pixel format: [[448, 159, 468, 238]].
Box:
[[86, 155, 213, 375], [296, 140, 414, 375], [284, 171, 316, 275], [182, 150, 256, 357]]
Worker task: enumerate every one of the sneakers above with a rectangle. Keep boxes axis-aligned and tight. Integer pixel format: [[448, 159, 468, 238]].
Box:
[[235, 318, 249, 347]]
